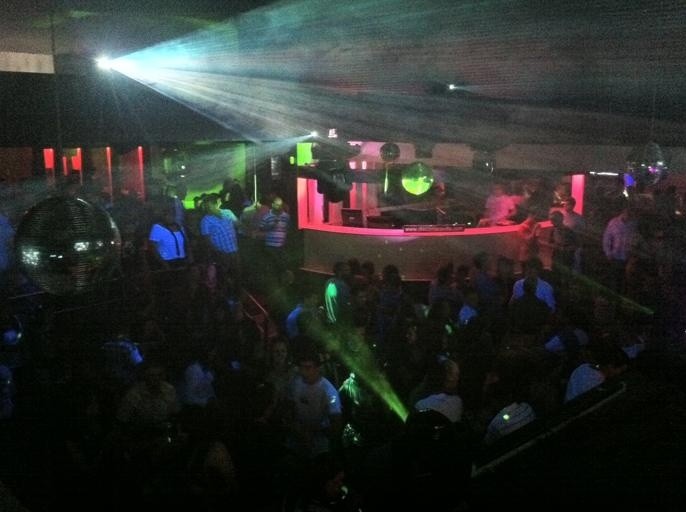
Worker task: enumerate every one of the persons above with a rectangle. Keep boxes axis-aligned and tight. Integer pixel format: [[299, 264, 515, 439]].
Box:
[[0, 177, 686, 512]]
